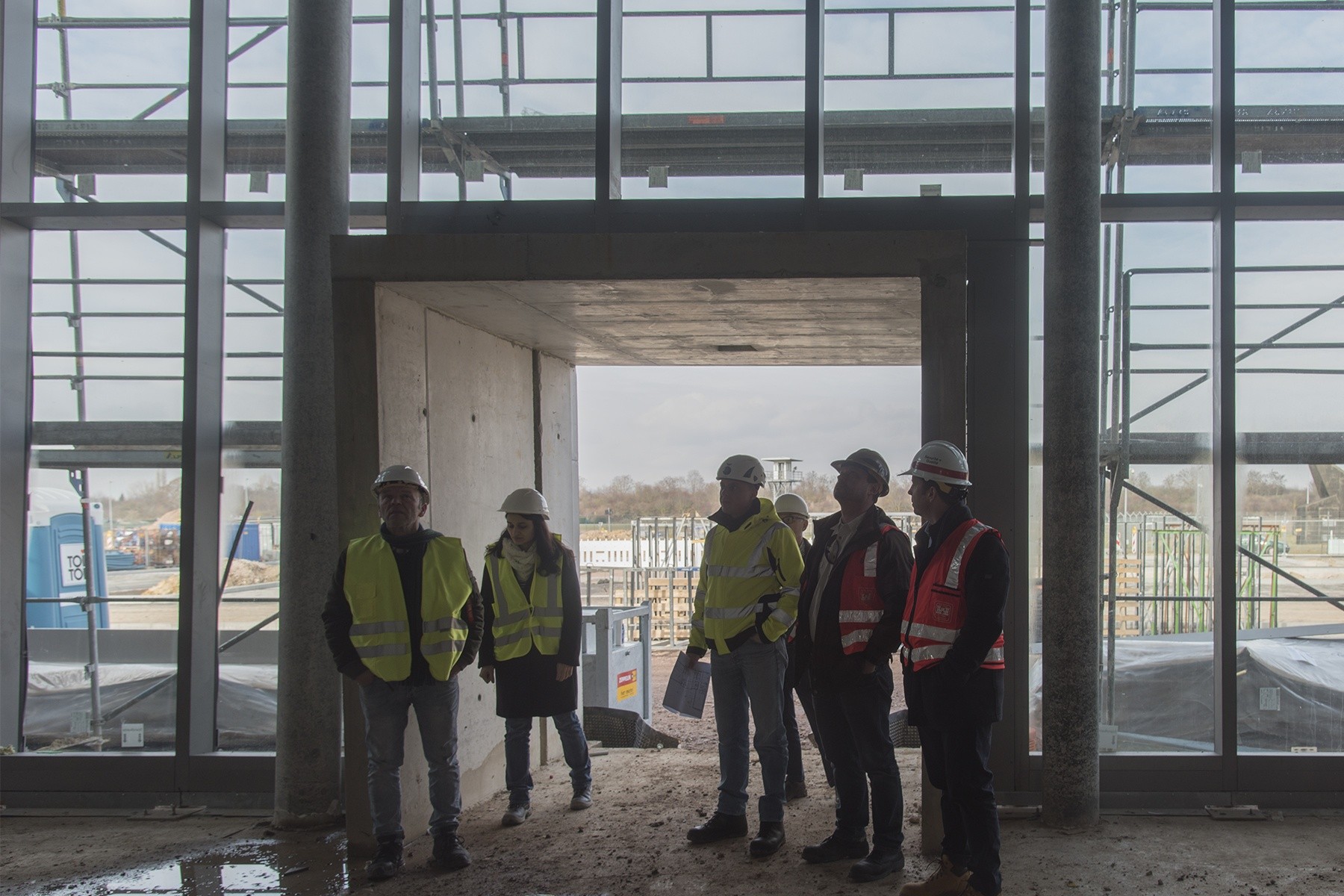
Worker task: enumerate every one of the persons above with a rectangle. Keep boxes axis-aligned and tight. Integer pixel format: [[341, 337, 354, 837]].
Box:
[[899, 441, 1011, 896], [322, 465, 485, 881], [684, 455, 804, 862], [774, 493, 835, 803], [162, 531, 175, 565], [478, 487, 592, 827], [796, 449, 914, 884]]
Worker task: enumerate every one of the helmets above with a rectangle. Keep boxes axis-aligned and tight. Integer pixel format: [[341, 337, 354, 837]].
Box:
[[716, 455, 765, 489], [897, 440, 972, 486], [830, 449, 891, 497], [774, 494, 810, 518], [370, 465, 430, 507], [497, 488, 550, 520]]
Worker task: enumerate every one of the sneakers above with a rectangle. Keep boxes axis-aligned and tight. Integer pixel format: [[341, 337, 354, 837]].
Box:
[[570, 784, 593, 809], [367, 844, 405, 880], [433, 835, 471, 869], [502, 801, 532, 825], [900, 854, 984, 896]]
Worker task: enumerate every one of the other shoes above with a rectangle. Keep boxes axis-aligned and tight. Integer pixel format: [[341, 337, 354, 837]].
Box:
[[785, 780, 807, 799], [687, 812, 748, 843], [849, 845, 904, 882], [802, 826, 869, 862], [750, 821, 786, 856]]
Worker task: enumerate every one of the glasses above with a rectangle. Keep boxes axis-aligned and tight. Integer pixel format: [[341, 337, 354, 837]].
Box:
[[782, 517, 805, 524]]
